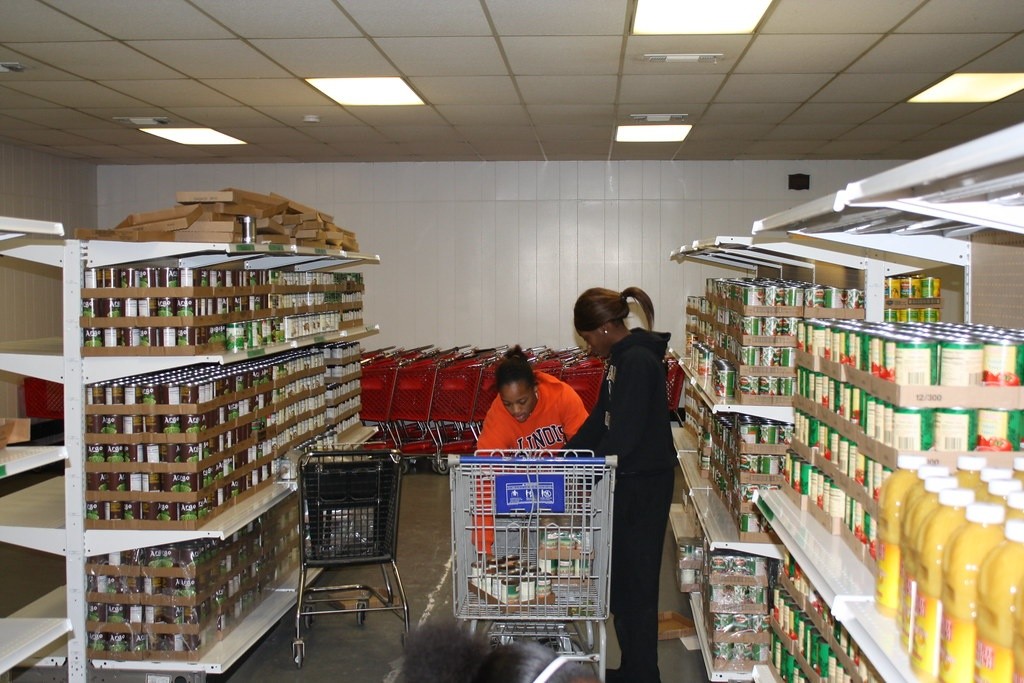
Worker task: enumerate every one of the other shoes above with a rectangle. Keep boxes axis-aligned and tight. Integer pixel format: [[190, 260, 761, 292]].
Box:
[[606, 668, 623, 683]]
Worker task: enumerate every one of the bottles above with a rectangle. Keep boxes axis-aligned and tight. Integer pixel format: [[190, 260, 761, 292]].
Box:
[[878, 442, 1024, 682]]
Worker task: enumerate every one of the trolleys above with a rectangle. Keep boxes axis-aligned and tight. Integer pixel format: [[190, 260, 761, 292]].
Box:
[[290, 441, 412, 670], [357, 346, 685, 476], [446, 449, 619, 683]]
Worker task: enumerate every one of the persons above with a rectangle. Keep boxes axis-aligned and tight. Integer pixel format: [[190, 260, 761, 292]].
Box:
[[399, 624, 602, 683], [471, 345, 588, 561], [560, 287, 678, 683]]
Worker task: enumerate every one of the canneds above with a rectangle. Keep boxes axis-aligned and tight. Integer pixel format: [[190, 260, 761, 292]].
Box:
[[83, 265, 364, 652], [685, 272, 1024, 683], [471, 531, 596, 604]]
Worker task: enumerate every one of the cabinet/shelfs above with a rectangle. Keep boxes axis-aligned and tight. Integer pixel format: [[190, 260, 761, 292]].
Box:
[[0, 217, 72, 683], [0, 238, 381, 683], [667, 123, 1023, 683]]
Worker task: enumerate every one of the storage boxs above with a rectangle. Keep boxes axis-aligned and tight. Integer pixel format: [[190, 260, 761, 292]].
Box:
[[658, 611, 697, 640], [74, 188, 360, 253], [0, 417, 31, 450]]
